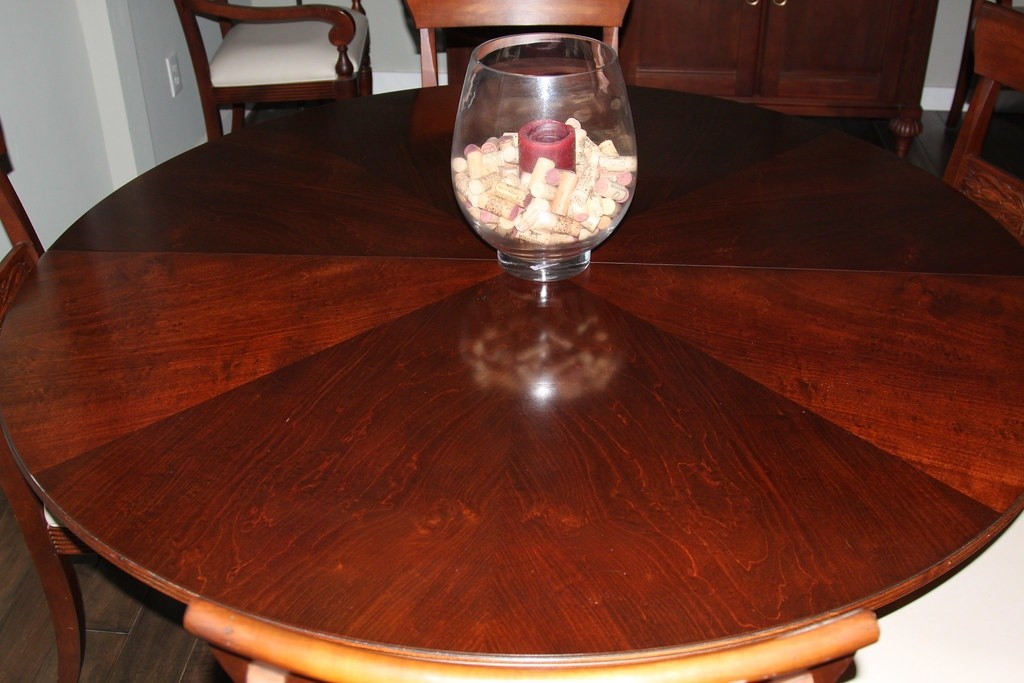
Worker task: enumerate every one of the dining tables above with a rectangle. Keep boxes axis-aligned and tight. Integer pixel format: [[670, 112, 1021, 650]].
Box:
[[0, 84, 1022, 672]]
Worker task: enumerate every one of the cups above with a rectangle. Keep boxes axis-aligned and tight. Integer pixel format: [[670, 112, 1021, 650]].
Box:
[[452, 34, 637, 283]]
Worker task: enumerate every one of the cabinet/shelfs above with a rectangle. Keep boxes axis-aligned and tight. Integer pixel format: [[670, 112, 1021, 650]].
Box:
[[619, 0, 941, 160]]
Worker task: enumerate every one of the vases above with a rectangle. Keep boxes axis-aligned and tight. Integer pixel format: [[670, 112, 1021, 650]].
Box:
[[447, 32, 639, 282]]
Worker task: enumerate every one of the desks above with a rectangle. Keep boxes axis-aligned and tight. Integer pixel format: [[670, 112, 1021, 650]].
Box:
[[401, 1, 628, 90]]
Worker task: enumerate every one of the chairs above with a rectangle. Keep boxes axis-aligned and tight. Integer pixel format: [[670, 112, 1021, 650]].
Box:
[[940, 5, 1023, 250], [171, 0, 377, 145], [947, 0, 1017, 130], [0, 121, 106, 678], [179, 592, 878, 683]]
